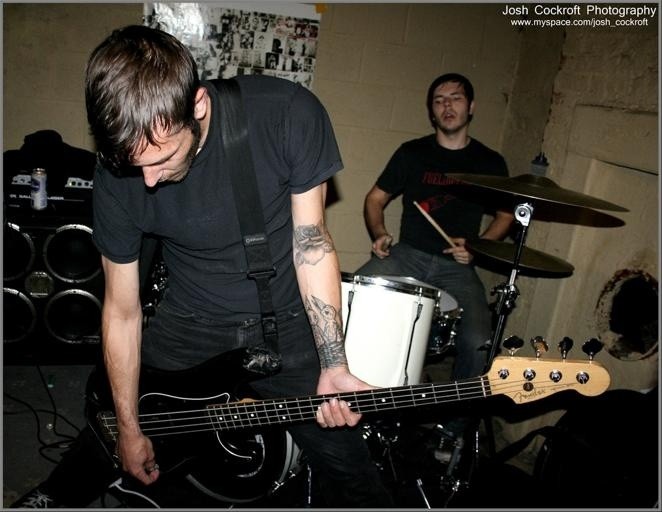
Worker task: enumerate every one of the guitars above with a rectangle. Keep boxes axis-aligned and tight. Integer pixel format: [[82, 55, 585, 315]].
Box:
[[85, 335, 611, 499]]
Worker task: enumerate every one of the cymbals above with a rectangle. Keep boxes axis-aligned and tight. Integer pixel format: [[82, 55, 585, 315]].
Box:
[[463, 239, 575, 272], [447, 174, 630, 213]]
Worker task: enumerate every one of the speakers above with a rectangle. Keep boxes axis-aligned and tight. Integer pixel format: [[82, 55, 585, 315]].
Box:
[[3, 188, 108, 365]]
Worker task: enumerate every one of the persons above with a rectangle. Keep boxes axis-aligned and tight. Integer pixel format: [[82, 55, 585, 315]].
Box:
[[10, 24, 384, 508], [362, 73, 518, 467]]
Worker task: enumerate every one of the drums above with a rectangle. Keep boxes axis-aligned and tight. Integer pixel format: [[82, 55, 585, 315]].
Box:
[[369, 274, 463, 363], [340, 271, 439, 388]]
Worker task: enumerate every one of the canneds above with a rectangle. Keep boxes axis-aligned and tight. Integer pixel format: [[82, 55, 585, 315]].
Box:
[[31, 168, 47, 210]]
